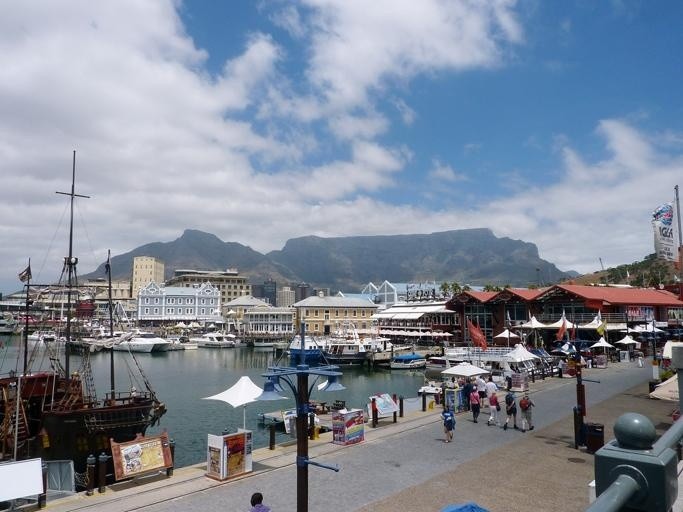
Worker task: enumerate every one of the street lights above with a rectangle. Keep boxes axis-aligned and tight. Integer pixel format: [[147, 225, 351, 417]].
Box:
[[253, 320, 346, 512], [551, 317, 601, 450]]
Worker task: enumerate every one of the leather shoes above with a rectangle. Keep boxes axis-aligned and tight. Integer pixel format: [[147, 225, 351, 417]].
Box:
[[504, 424, 534, 432]]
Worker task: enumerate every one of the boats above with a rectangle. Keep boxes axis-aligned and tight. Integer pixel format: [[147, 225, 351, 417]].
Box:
[[29, 326, 236, 353], [287, 322, 427, 370]]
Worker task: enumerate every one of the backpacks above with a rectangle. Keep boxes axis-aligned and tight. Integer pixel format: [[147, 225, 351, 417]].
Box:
[[520, 397, 529, 409]]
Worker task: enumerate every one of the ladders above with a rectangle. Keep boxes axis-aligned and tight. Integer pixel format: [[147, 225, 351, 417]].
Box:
[[3, 387, 30, 442]]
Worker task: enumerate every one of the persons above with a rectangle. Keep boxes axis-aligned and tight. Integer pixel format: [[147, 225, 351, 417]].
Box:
[[556, 357, 565, 378], [501, 391, 518, 432], [518, 394, 535, 433], [246, 492, 273, 512], [468, 385, 481, 424], [438, 375, 498, 412], [486, 390, 499, 427], [439, 406, 455, 444]]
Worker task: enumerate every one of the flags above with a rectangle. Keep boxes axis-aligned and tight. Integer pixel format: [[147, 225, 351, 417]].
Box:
[[648, 200, 680, 265], [16, 265, 33, 283], [466, 317, 484, 353], [475, 320, 486, 349]]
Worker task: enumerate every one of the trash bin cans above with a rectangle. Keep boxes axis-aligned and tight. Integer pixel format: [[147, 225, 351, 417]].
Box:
[[649, 382, 660, 399], [585, 423, 604, 453], [434, 395, 439, 404]]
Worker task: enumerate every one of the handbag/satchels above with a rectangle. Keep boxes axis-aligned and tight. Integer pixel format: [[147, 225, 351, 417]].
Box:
[[496, 405, 501, 411]]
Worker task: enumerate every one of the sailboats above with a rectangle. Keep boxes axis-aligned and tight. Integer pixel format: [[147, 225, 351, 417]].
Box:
[[0, 150, 166, 469]]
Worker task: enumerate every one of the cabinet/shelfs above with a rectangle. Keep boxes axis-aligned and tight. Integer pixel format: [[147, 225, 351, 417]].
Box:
[[445, 388, 469, 414], [332, 409, 365, 446], [207, 430, 253, 481]]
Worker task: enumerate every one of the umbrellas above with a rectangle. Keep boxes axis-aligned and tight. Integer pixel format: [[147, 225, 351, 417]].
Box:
[[197, 375, 291, 429], [647, 378, 680, 403], [490, 314, 666, 373], [652, 372, 678, 387]]
